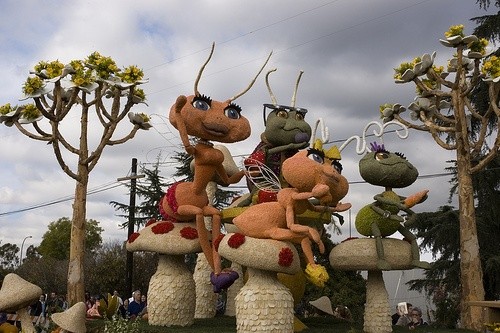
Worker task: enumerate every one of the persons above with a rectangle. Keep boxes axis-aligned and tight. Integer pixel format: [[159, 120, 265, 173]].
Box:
[[85, 289, 147, 320], [392, 302, 413, 326], [30, 292, 69, 317], [405, 307, 429, 331]]
